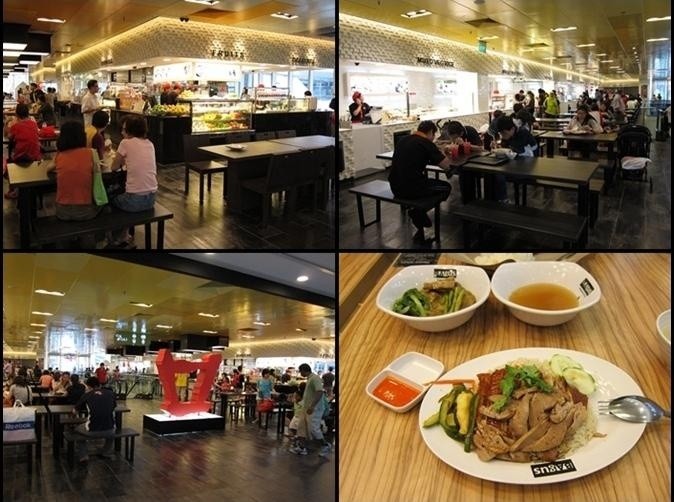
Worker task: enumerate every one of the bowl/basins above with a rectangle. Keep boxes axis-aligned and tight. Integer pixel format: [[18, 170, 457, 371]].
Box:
[[375, 264, 490, 333], [494, 148, 511, 159], [490, 261, 602, 326]]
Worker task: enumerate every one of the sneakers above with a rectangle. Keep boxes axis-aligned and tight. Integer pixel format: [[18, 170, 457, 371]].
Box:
[[408, 207, 433, 230], [319, 445, 332, 456], [291, 445, 308, 456]]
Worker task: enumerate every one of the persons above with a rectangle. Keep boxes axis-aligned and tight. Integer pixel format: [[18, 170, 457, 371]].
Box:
[[104, 114, 159, 248], [348, 91, 369, 124], [83, 110, 109, 165], [2, 82, 60, 138], [387, 120, 452, 230], [53, 121, 112, 250], [1, 358, 335, 463], [442, 83, 670, 214], [140, 80, 312, 115], [79, 80, 102, 134], [4, 103, 42, 200]]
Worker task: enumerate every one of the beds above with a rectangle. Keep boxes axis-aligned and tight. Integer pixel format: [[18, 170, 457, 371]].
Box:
[[1, 95, 336, 250]]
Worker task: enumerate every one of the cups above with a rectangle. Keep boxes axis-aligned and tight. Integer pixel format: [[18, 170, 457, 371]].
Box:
[[656, 309, 670, 372], [450, 141, 471, 161]]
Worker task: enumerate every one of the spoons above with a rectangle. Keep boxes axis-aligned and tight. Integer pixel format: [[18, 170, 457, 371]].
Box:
[[608, 394, 670, 423]]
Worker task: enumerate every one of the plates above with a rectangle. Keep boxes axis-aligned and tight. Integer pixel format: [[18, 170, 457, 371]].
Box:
[[365, 351, 444, 413], [226, 144, 245, 151], [417, 347, 647, 485]]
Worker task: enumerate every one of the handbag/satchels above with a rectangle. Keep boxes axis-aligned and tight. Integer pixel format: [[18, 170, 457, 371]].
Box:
[[19, 402, 32, 407], [89, 149, 108, 206]]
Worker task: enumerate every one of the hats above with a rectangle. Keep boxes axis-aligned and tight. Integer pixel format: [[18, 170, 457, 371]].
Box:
[[36, 94, 46, 102], [173, 84, 182, 90], [352, 92, 362, 100], [161, 83, 171, 89]]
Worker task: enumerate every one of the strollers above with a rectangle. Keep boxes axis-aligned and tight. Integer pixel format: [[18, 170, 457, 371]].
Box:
[[612, 124, 654, 193]]
